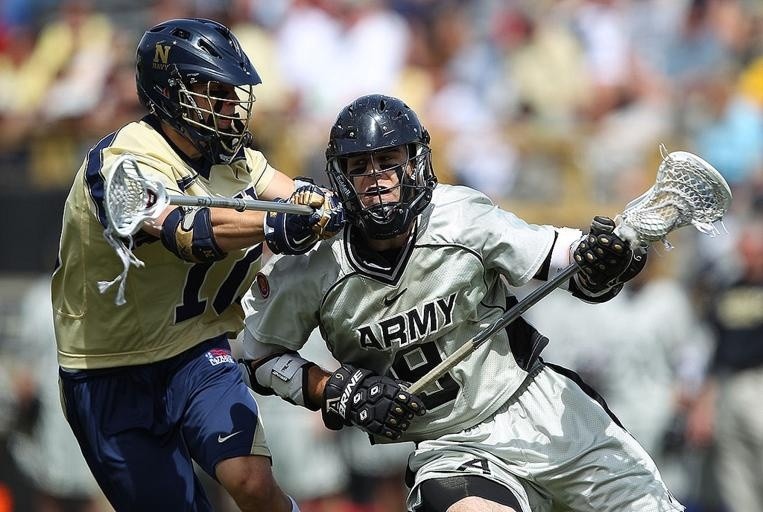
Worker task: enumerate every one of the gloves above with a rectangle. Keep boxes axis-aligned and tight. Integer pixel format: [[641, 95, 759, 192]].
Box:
[[262, 178, 347, 256], [570, 212, 649, 305], [319, 361, 428, 442]]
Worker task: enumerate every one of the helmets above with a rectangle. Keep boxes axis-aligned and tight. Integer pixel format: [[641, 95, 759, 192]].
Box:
[[133, 16, 263, 167], [323, 93, 439, 242]]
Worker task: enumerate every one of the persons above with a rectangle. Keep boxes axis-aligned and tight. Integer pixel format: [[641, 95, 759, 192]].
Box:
[[0, 1, 150, 512], [236, 1, 430, 509], [50, 17, 349, 510], [234, 94, 684, 510], [430, 2, 763, 512]]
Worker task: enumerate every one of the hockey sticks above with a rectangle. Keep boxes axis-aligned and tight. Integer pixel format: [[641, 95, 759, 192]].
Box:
[[105, 157, 314, 236], [408, 152, 733, 392]]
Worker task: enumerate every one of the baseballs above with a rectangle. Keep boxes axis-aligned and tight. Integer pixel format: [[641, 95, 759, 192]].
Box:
[[639, 213, 667, 241]]
